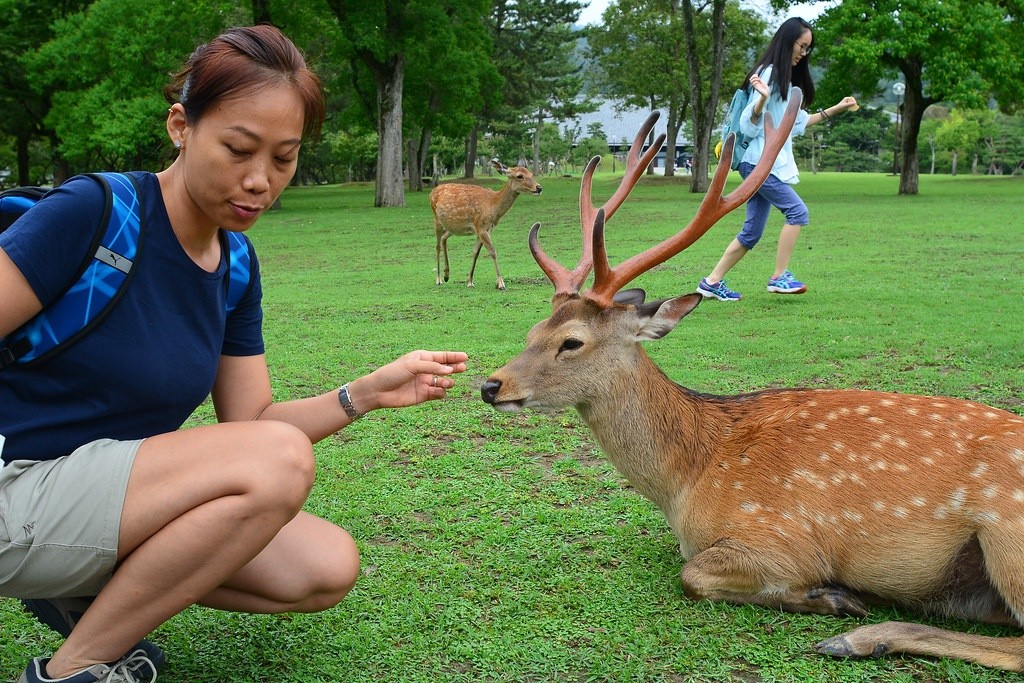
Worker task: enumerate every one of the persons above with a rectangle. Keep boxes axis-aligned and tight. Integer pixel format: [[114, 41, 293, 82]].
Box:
[[674, 160, 677, 172], [685, 158, 693, 175], [549, 160, 557, 170], [696, 17, 856, 300], [0, 24, 469, 683]]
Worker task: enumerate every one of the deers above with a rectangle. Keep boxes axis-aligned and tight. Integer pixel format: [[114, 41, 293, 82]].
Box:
[[428, 85, 1024, 672]]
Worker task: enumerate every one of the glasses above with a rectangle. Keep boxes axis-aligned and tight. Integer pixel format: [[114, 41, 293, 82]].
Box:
[[795, 41, 811, 55]]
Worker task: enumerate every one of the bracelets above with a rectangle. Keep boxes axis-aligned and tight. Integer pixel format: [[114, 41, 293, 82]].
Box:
[[751, 105, 762, 120], [338, 382, 367, 421], [821, 110, 830, 119]]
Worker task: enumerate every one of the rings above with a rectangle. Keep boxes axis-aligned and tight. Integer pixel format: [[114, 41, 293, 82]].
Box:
[[434, 375, 438, 387]]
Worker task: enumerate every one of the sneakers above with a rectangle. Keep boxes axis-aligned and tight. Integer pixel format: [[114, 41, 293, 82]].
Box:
[[768, 270, 806, 294], [18, 649, 159, 683], [17, 596, 164, 680], [696, 277, 741, 302]]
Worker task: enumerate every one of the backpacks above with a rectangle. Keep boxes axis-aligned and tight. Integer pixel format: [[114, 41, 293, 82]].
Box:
[[718, 65, 780, 171]]
